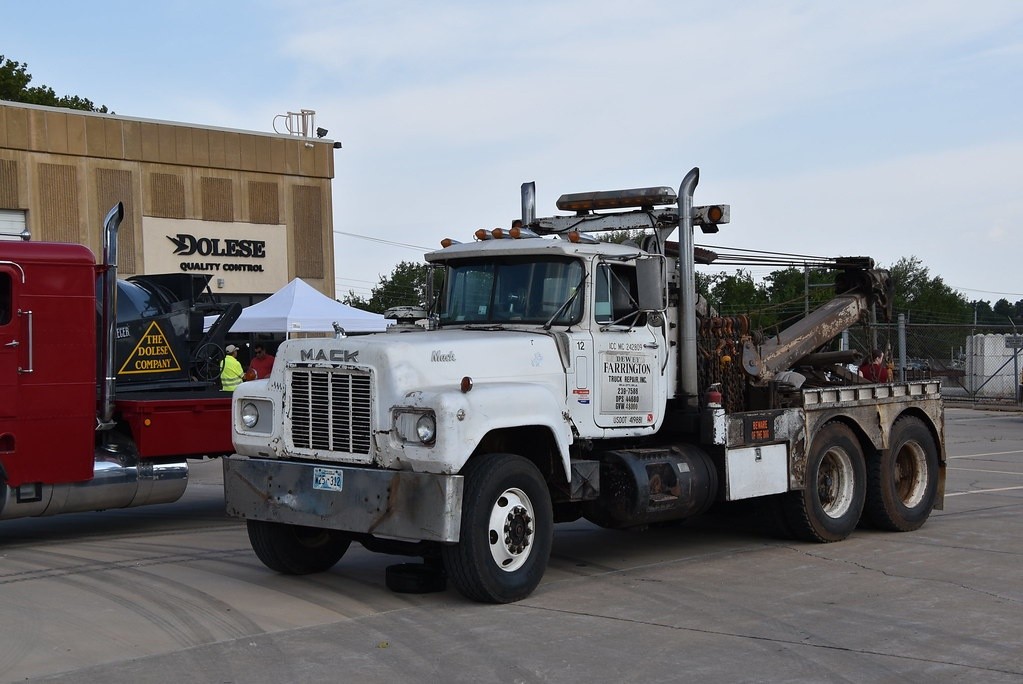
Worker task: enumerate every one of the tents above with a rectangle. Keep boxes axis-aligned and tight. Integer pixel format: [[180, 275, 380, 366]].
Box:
[[203, 275, 388, 344]]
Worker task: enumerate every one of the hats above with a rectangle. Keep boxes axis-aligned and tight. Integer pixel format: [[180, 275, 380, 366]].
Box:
[[226, 345, 239, 354]]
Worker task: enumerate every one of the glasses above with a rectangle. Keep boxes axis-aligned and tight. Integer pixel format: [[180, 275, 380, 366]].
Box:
[[255, 350, 262, 355]]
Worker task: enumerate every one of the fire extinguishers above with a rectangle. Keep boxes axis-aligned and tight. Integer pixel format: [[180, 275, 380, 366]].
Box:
[[706, 383, 723, 408]]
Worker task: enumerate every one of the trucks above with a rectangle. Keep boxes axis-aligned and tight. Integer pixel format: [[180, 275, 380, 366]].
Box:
[[222, 168, 947, 605], [0, 200, 234, 532]]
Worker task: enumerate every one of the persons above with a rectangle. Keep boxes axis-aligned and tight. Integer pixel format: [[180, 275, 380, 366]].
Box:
[[219, 345, 247, 392], [245, 342, 275, 382], [857, 347, 889, 382]]
